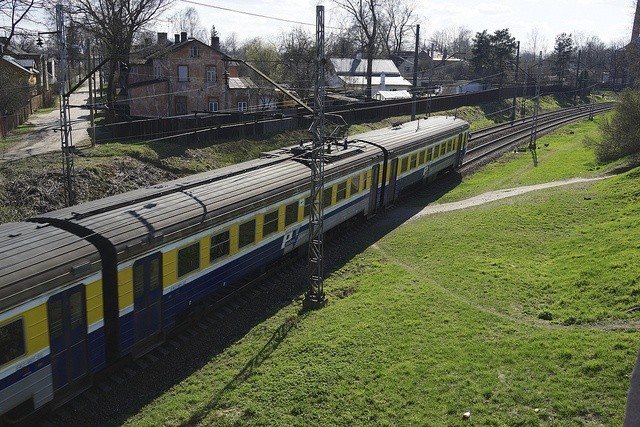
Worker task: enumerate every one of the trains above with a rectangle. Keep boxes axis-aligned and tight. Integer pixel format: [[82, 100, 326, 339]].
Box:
[[1, 116, 470, 427]]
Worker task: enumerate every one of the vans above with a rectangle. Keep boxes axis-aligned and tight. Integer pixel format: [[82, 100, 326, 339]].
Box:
[[275, 88, 302, 108]]
